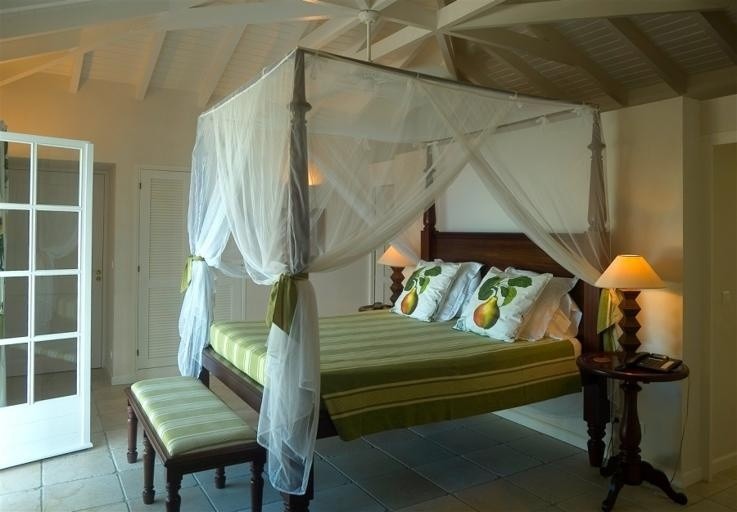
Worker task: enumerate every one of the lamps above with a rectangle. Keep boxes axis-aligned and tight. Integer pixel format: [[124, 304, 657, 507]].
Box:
[[376, 242, 416, 306], [593, 252, 664, 357]]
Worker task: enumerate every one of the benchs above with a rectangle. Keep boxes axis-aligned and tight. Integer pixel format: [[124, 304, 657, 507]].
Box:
[[123, 376, 266, 512]]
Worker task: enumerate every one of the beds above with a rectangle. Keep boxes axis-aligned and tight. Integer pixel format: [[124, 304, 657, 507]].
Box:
[[186, 231, 607, 510]]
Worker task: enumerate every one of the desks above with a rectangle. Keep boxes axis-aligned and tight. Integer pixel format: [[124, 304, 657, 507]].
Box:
[[574, 351, 690, 512]]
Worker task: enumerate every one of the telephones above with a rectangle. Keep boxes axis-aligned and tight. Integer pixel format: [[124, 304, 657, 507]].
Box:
[[614, 352, 683, 373]]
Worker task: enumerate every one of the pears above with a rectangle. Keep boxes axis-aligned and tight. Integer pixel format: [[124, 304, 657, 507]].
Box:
[[401, 279, 419, 314], [473, 288, 500, 329]]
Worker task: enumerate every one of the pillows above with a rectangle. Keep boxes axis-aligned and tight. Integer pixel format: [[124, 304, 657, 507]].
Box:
[[387, 257, 582, 344]]
